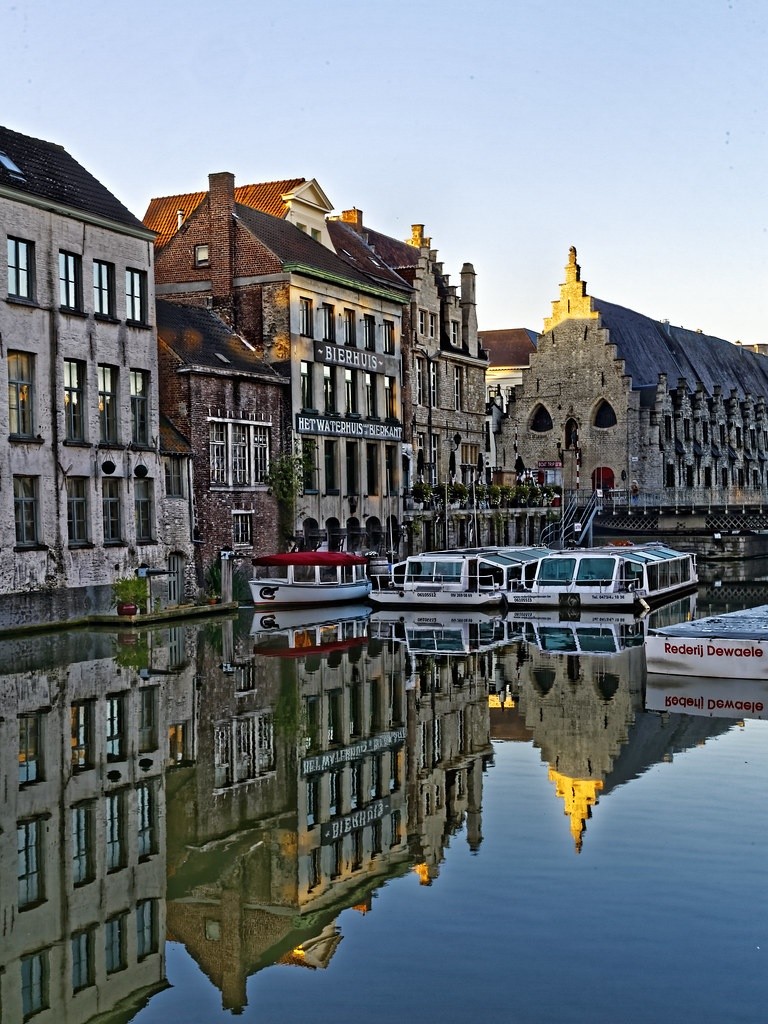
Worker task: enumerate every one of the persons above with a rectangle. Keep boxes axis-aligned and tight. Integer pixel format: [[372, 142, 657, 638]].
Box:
[[631, 481, 640, 506]]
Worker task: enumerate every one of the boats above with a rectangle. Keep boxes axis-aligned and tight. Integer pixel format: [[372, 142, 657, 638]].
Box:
[[248, 597, 373, 658], [646, 605, 768, 680], [644, 674, 768, 720], [364, 469, 397, 574], [247, 552, 372, 606], [370, 592, 698, 656], [368, 544, 699, 610]]
